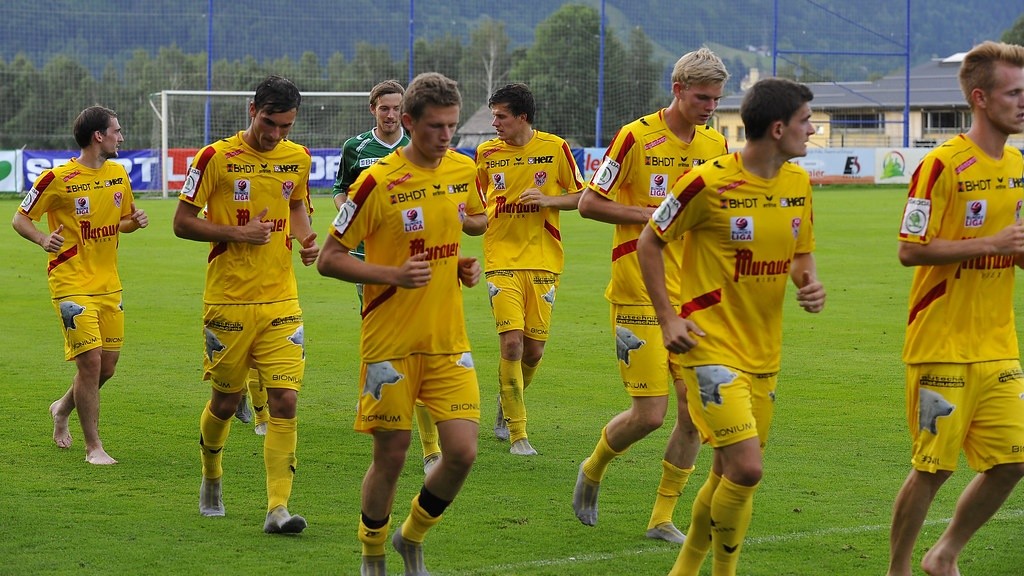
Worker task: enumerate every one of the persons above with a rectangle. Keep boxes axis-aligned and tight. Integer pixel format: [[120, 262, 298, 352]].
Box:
[[573, 48, 730, 545], [475, 83, 586, 455], [636, 78, 826, 576], [172, 75, 320, 535], [331, 81, 410, 304], [316, 72, 487, 576], [12, 107, 148, 464], [885, 40, 1024, 576]]
